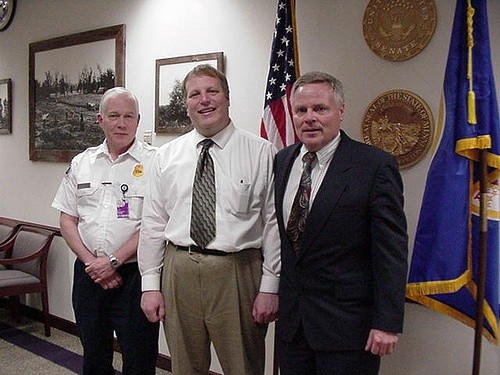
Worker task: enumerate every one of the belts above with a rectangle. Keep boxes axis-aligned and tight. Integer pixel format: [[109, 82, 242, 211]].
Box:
[[169, 241, 228, 257]]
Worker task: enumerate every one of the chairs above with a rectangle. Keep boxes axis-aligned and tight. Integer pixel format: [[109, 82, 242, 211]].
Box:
[[0, 217, 60, 338]]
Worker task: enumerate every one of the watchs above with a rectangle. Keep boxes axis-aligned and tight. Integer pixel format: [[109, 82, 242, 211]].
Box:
[[109, 254, 120, 267]]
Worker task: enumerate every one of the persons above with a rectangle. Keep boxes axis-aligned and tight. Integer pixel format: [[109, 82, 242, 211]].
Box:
[[51, 87, 159, 375], [273, 71, 409, 375], [136, 64, 282, 375]]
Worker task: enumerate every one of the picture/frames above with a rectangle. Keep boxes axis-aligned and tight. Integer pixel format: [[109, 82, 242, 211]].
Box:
[[0, 79, 12, 135], [28, 22, 128, 162], [154, 51, 228, 133]]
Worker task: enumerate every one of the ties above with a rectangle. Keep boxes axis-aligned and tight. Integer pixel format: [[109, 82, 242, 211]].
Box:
[[190, 139, 217, 250], [287, 152, 317, 260]]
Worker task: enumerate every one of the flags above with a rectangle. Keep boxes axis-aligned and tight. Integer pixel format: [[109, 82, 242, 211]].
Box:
[[260, 0, 300, 151], [405, 0, 500, 345]]
